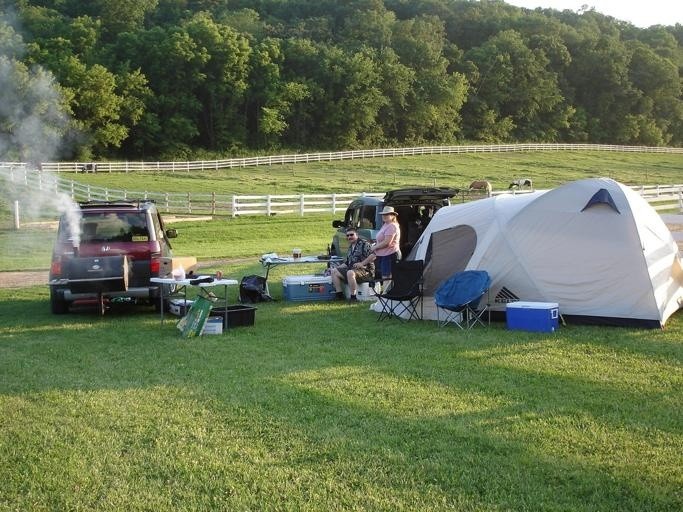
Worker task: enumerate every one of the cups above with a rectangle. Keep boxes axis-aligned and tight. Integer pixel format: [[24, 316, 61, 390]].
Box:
[[293, 249, 301, 261]]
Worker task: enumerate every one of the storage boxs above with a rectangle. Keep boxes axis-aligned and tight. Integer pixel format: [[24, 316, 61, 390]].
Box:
[[506, 301, 559, 333], [282, 274, 381, 302], [159, 256, 197, 279], [203, 316, 223, 336]]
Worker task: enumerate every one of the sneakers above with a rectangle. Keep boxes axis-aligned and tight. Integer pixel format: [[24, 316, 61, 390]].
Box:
[[349, 298, 357, 302], [334, 295, 345, 301]]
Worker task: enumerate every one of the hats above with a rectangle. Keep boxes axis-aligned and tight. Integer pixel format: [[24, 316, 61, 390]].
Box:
[[378, 205, 399, 216]]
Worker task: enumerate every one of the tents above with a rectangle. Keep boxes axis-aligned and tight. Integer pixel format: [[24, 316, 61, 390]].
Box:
[[372, 175, 682, 327]]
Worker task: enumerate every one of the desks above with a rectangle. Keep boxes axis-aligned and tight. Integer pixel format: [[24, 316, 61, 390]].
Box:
[[150, 276, 237, 335], [259, 255, 344, 296]]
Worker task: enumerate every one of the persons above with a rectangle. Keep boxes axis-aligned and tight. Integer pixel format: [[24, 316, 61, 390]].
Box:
[[370, 206, 401, 294], [331, 226, 374, 306]]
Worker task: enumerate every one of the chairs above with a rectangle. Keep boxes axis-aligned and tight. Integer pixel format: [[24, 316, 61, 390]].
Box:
[[368, 260, 492, 332]]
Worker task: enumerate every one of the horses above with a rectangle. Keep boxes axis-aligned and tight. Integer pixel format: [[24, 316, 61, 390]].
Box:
[[508, 178, 532, 190], [468, 181, 492, 194]]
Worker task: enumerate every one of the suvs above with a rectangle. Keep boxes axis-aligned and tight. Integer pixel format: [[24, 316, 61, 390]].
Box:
[[48, 200, 177, 314], [331, 186, 461, 280]]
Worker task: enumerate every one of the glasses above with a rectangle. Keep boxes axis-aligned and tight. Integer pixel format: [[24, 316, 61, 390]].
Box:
[[347, 234, 354, 236]]
